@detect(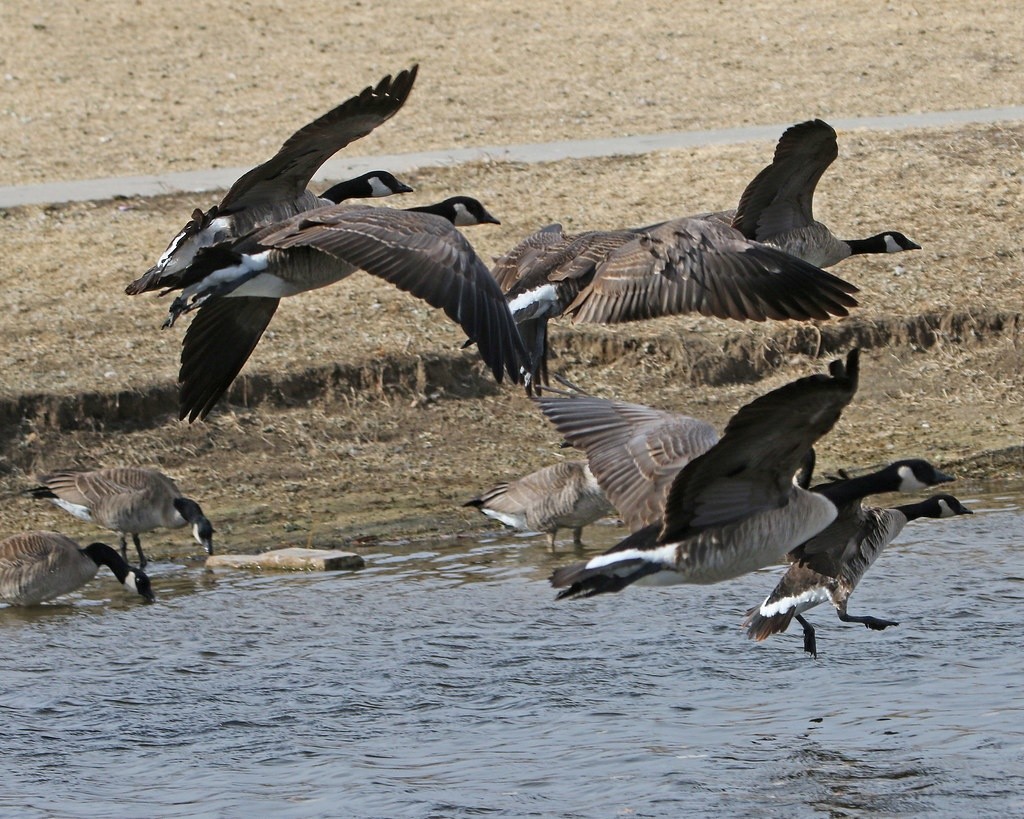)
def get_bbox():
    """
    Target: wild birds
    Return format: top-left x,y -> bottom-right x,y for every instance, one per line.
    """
175,195 -> 540,426
123,61 -> 423,298
1,530 -> 156,610
14,464 -> 216,571
459,115 -> 921,400
457,345 -> 973,660
